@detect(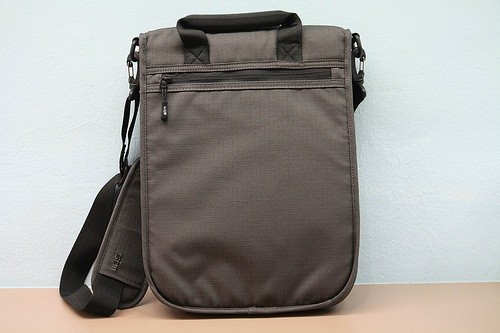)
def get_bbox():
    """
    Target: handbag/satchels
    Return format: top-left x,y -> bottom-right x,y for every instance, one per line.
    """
59,10 -> 366,313
